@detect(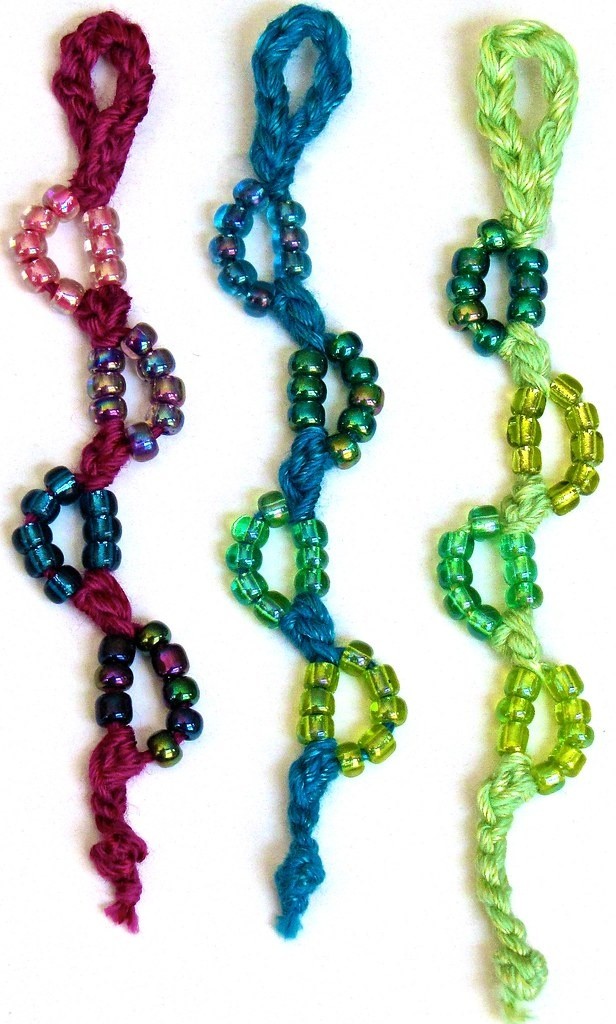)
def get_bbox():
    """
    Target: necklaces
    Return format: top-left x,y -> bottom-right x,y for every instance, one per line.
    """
4,9 -> 202,935
209,5 -> 407,937
435,16 -> 603,1018
